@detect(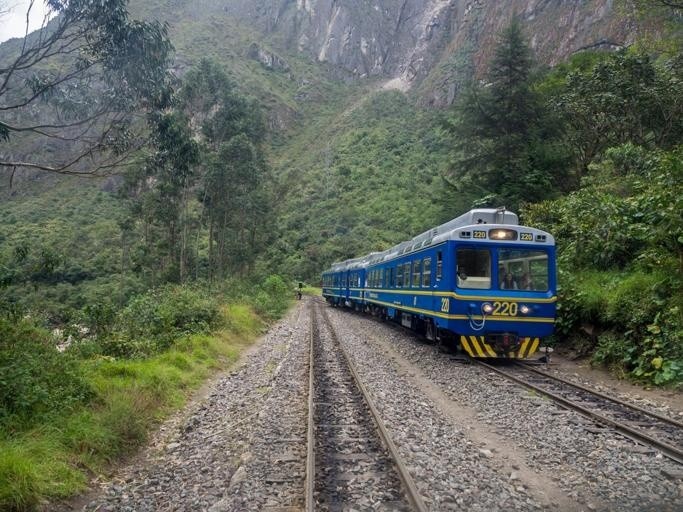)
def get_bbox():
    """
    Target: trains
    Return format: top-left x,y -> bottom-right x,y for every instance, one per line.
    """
320,203 -> 560,366
